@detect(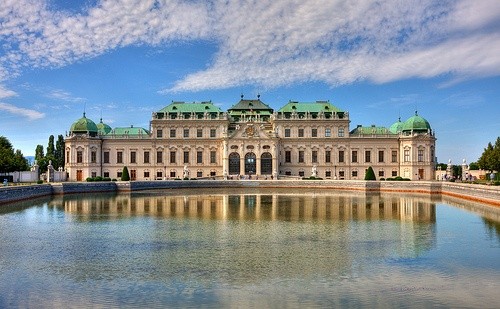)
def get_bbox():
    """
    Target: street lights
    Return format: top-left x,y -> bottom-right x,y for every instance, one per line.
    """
99,138 -> 104,178
